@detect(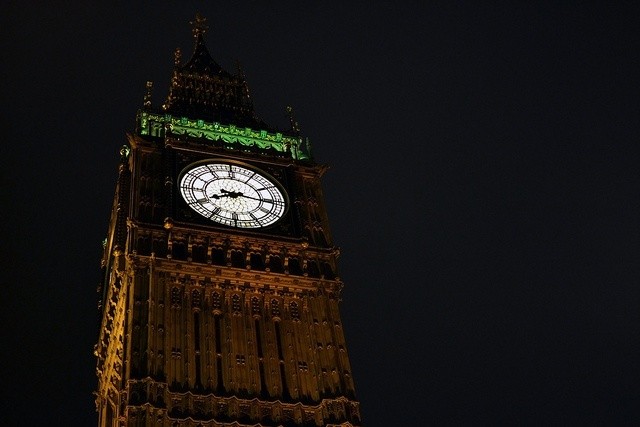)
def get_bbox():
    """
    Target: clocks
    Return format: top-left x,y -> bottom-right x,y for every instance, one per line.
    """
178,158 -> 288,231
